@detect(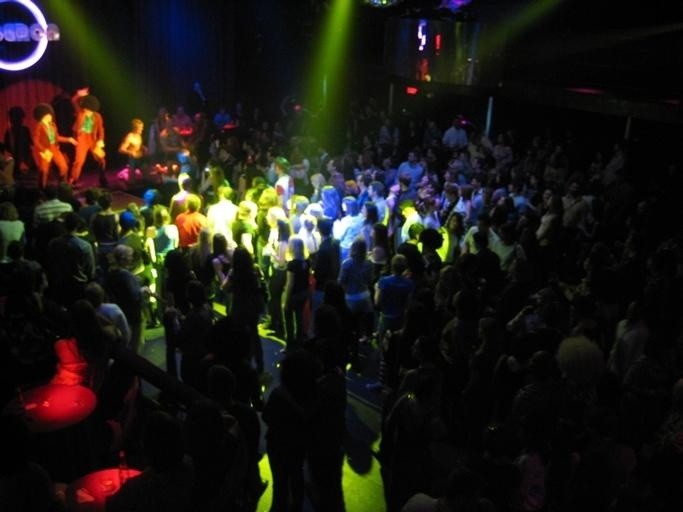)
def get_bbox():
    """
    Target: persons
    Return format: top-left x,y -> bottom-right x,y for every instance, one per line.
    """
0,88 -> 682,512
